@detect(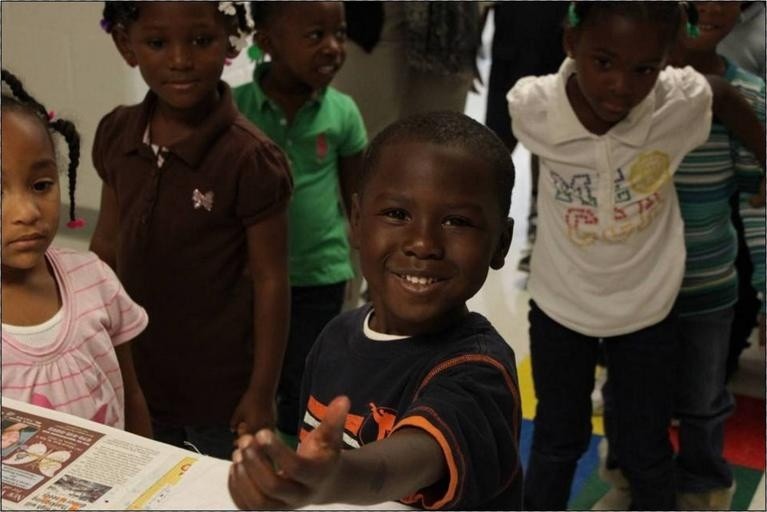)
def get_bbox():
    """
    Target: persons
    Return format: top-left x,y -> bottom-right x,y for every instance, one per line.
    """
468,1 -> 569,272
1,67 -> 155,441
227,110 -> 524,511
230,1 -> 371,435
86,1 -> 294,460
